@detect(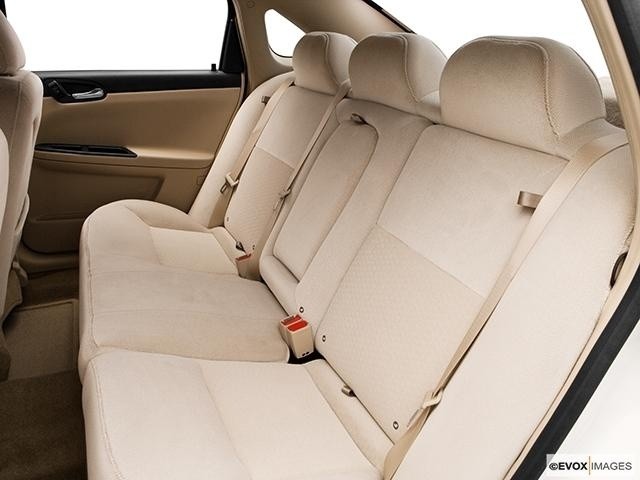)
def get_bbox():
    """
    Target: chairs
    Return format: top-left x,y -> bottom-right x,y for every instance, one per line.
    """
0,12 -> 43,382
